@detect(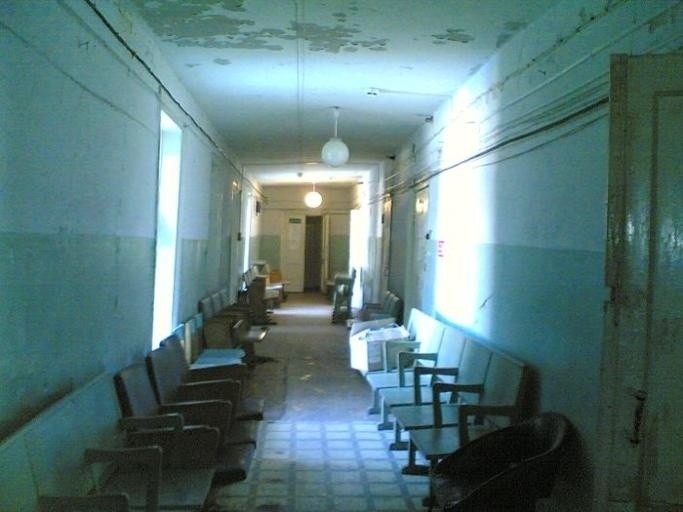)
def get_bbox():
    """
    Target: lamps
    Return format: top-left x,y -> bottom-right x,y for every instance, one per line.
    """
304,181 -> 323,210
321,105 -> 350,168
